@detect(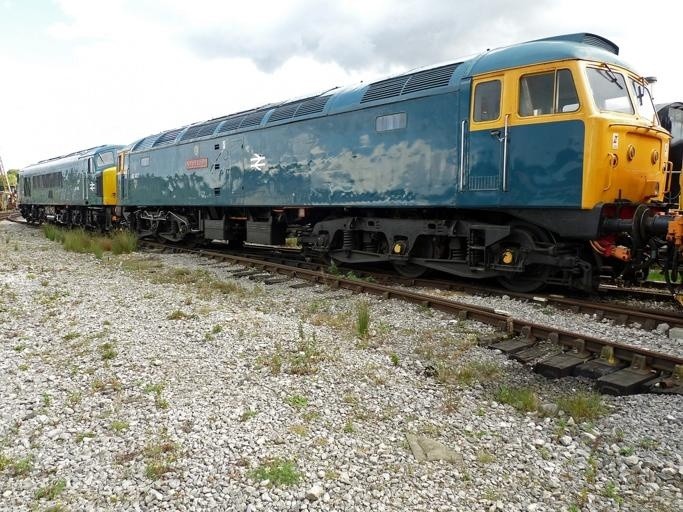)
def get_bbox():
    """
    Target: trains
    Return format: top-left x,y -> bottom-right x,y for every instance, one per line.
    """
16,32 -> 683,299
